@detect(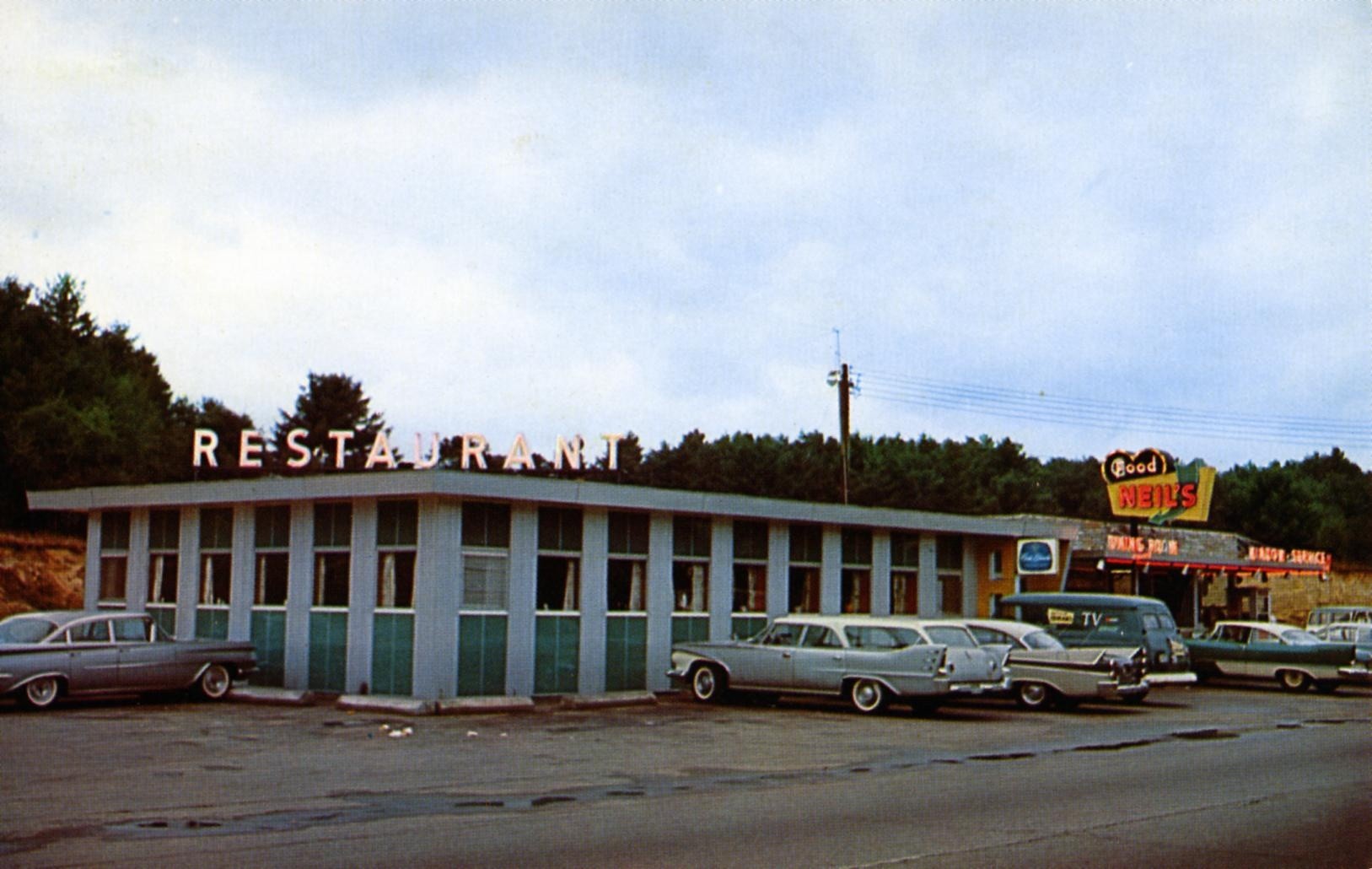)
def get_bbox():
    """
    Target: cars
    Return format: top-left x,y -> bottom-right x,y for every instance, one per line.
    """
958,617 -> 1149,709
667,612 -> 1014,718
0,610 -> 257,713
1305,605 -> 1372,643
998,591 -> 1197,705
1182,619 -> 1372,695
